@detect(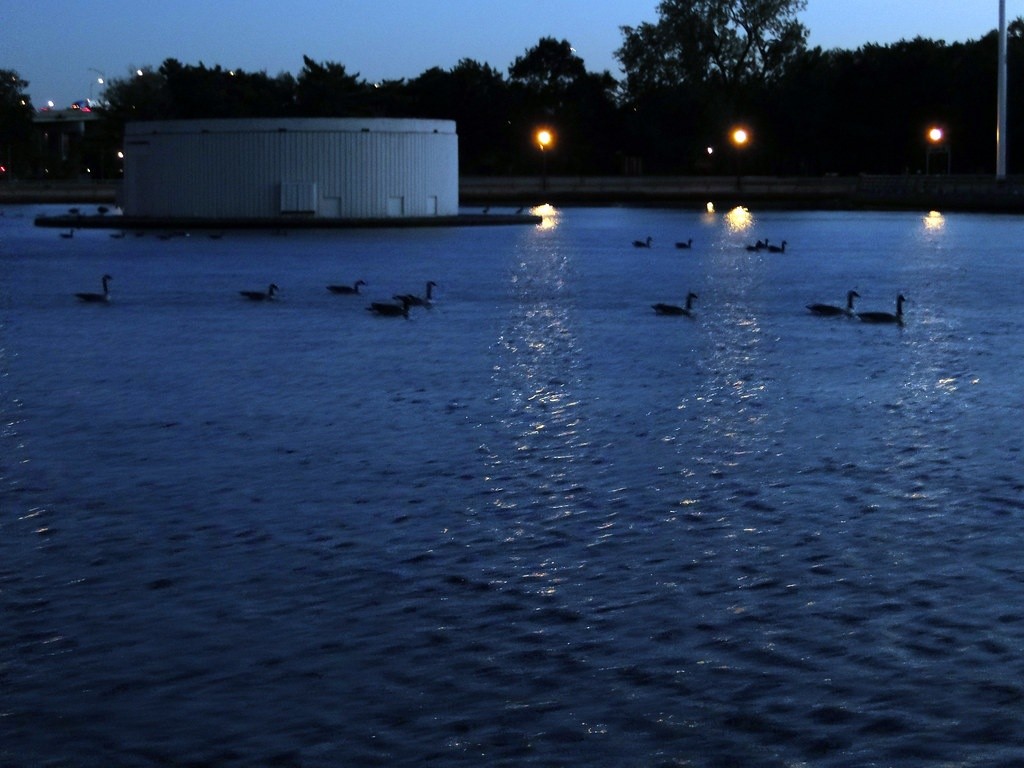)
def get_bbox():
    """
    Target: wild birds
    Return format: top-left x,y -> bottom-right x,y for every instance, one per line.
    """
631,236 -> 653,249
675,238 -> 693,250
745,237 -> 788,254
72,274 -> 114,303
325,280 -> 366,294
365,280 -> 439,314
59,228 -> 75,239
805,289 -> 861,316
109,229 -> 225,241
237,283 -> 279,302
650,292 -> 698,317
856,294 -> 906,324
